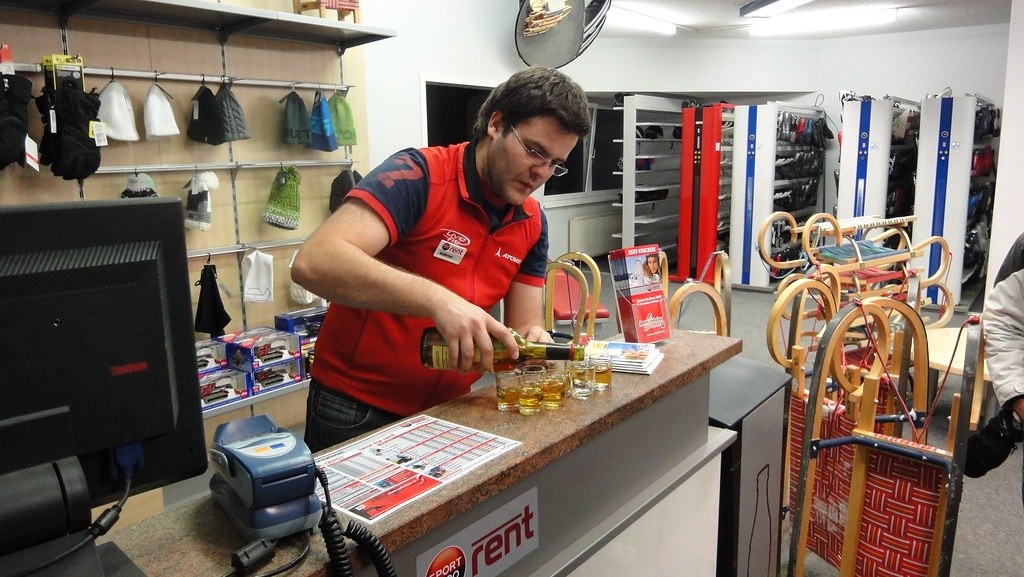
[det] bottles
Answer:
[420,327,584,372]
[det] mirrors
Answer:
[514,0,612,70]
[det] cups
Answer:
[496,354,612,416]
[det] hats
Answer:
[184,171,219,230]
[262,166,302,230]
[215,85,251,141]
[328,92,357,146]
[144,84,180,142]
[307,90,338,152]
[279,92,312,145]
[187,86,225,146]
[96,82,140,142]
[193,266,232,333]
[329,170,363,213]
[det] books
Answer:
[583,241,674,376]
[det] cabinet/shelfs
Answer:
[908,86,1001,313]
[611,94,693,264]
[668,103,735,287]
[728,100,826,293]
[834,89,921,291]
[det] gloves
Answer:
[0,74,100,180]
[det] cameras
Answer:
[960,406,1024,478]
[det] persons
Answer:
[962,228,1024,479]
[642,253,659,285]
[288,66,593,455]
[980,263,1024,504]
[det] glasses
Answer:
[510,124,568,177]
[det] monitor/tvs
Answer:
[0,197,209,577]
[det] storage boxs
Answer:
[226,357,304,397]
[274,307,329,345]
[197,368,252,411]
[211,326,301,372]
[195,339,229,376]
[300,342,318,380]
